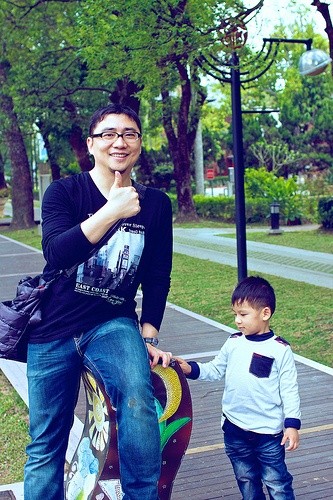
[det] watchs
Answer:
[142,336,159,347]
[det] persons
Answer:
[24,104,172,500]
[172,275,301,500]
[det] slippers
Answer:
[90,131,141,141]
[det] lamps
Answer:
[270,199,281,230]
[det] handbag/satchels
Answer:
[1,272,50,364]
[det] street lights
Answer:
[190,1,332,283]
